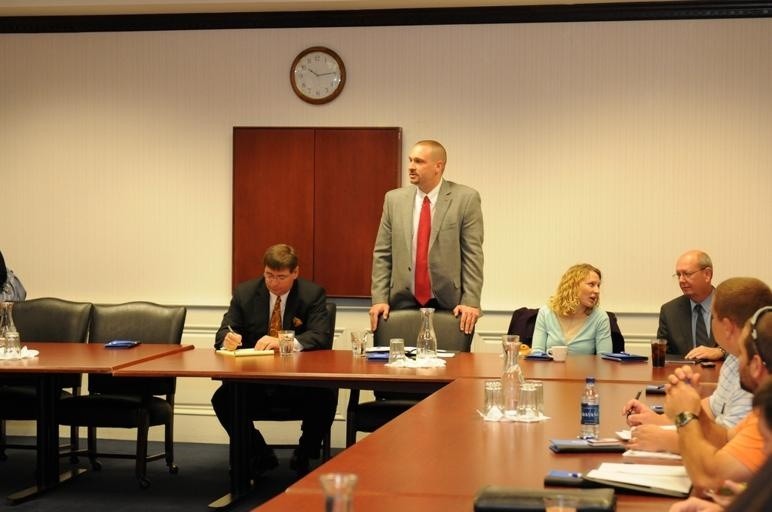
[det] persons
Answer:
[620,277,772,451]
[208,242,340,487]
[531,263,613,357]
[661,307,772,499]
[666,379,772,511]
[366,140,484,336]
[657,250,730,359]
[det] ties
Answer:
[415,196,432,306]
[695,304,709,347]
[269,296,282,352]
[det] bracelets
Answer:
[718,343,727,358]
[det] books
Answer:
[474,486,619,512]
[523,351,553,361]
[664,354,704,365]
[215,346,275,359]
[543,469,626,493]
[645,384,666,394]
[105,339,142,349]
[589,459,695,502]
[547,436,625,453]
[365,343,458,362]
[602,349,649,363]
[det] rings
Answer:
[631,436,637,443]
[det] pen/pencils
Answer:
[228,325,243,347]
[627,390,641,415]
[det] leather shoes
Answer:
[289,456,324,474]
[228,448,279,477]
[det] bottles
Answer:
[579,374,601,441]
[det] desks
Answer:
[251,375,719,512]
[1,338,194,507]
[114,345,726,509]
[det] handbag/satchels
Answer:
[0,270,26,302]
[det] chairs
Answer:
[509,309,625,352]
[228,304,338,485]
[344,306,477,469]
[66,295,187,481]
[0,295,95,480]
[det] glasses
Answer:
[672,267,705,279]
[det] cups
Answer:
[546,345,570,363]
[515,380,547,418]
[0,300,16,359]
[500,335,527,419]
[3,332,21,359]
[388,338,405,367]
[650,338,667,369]
[320,475,358,512]
[415,307,440,364]
[276,328,295,357]
[349,330,369,357]
[480,379,506,419]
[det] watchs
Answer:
[674,411,698,430]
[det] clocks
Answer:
[289,46,347,106]
